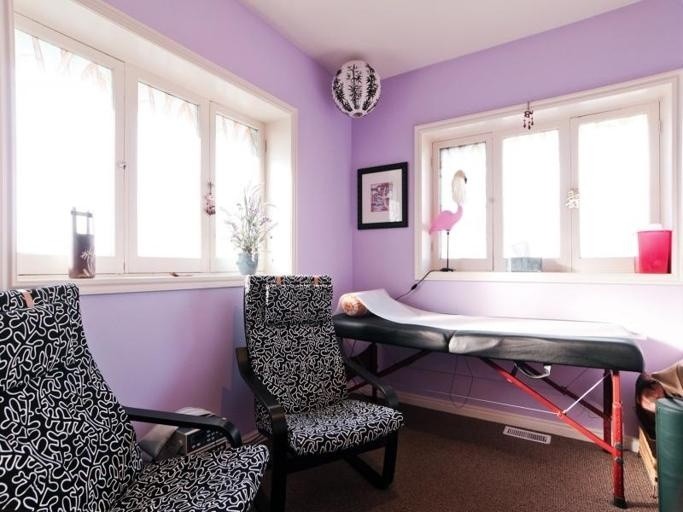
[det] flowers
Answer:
[224,184,276,262]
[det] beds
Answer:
[332,313,643,508]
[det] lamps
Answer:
[332,59,381,119]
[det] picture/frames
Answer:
[358,162,408,230]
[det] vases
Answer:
[238,254,257,274]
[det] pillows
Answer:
[0,302,75,393]
[341,295,371,317]
[265,285,333,325]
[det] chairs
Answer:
[236,274,404,512]
[0,283,270,512]
